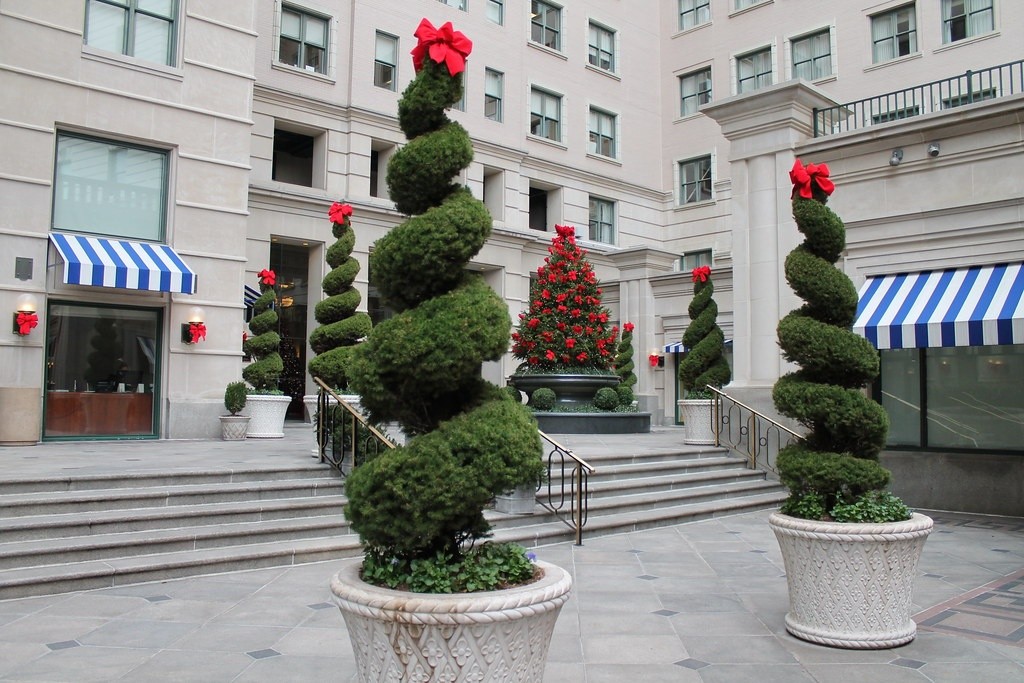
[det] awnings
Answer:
[45,234,198,296]
[662,339,735,353]
[844,259,1024,350]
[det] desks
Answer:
[43,392,152,436]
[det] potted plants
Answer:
[217,381,251,441]
[84,302,125,392]
[302,201,372,457]
[327,16,572,682]
[766,156,934,650]
[676,264,730,445]
[240,268,292,437]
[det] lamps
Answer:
[12,293,37,335]
[181,306,206,344]
[650,348,664,368]
